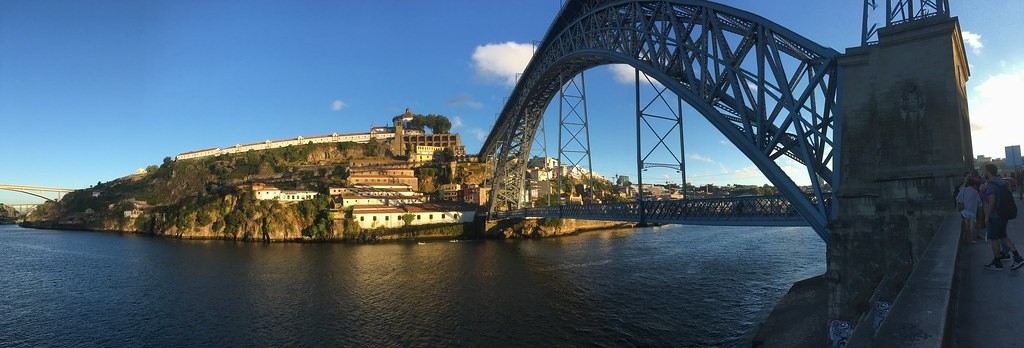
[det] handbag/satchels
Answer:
[957,202,964,211]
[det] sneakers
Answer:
[984,262,1003,271]
[1010,256,1024,270]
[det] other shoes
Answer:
[967,239,978,244]
[1000,251,1010,261]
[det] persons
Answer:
[953,169,1024,261]
[813,195,832,206]
[984,164,1024,271]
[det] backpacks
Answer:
[986,180,1017,219]
